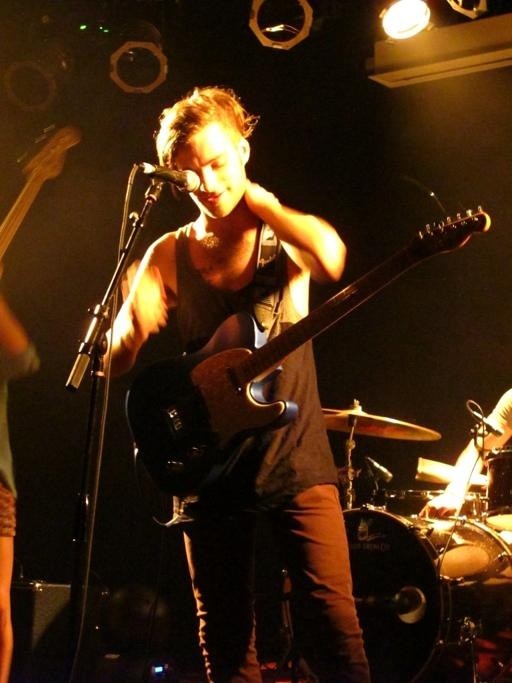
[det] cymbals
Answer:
[321,405,442,441]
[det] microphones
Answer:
[474,411,504,439]
[137,161,199,193]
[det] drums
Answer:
[282,503,512,683]
[484,446,512,532]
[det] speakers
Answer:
[11,580,74,683]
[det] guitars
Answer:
[124,206,492,495]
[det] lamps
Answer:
[0,19,169,115]
[247,1,313,53]
[366,0,433,40]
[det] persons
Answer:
[418,388,512,521]
[98,80,379,683]
[0,262,47,682]
